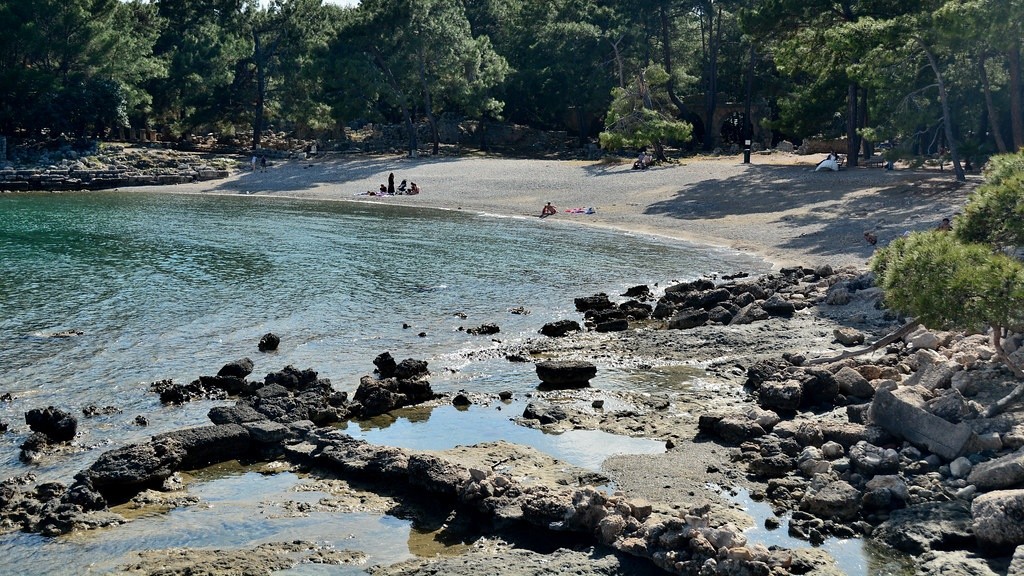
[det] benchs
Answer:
[834,158,844,171]
[865,155,884,168]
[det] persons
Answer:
[252,156,257,175]
[631,152,653,170]
[815,150,840,172]
[260,155,268,173]
[397,179,408,195]
[863,231,877,245]
[938,218,953,231]
[543,202,559,214]
[407,182,420,195]
[380,184,387,193]
[388,173,395,195]
